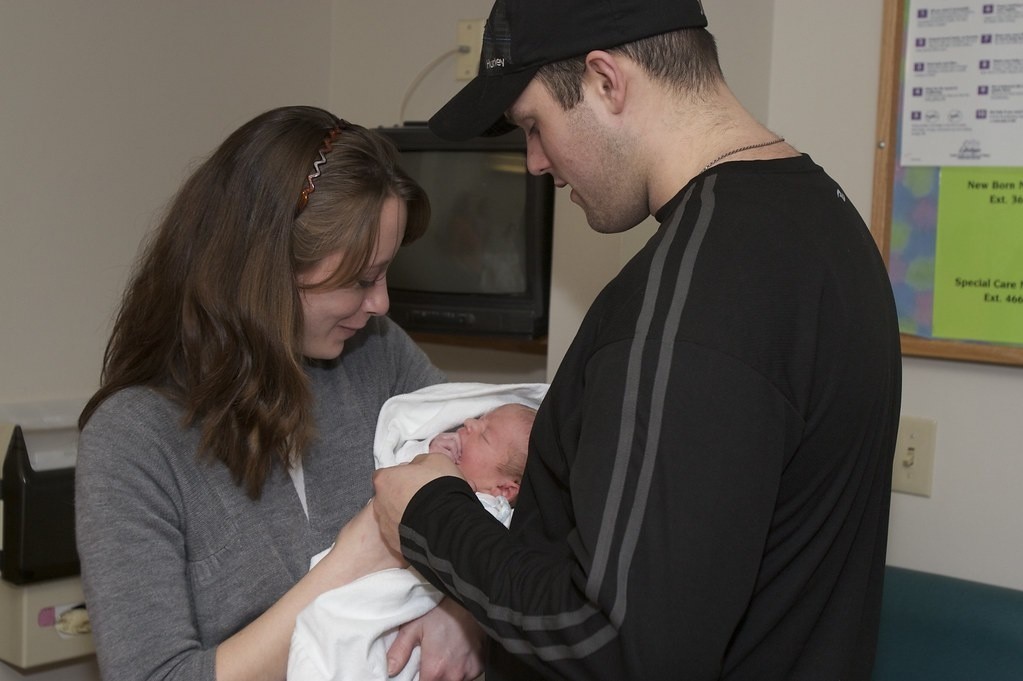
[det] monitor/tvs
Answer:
[367,120,555,340]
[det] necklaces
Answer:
[699,138,785,174]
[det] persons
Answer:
[287,402,538,681]
[371,0,901,681]
[73,105,491,680]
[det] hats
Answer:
[428,0,707,142]
